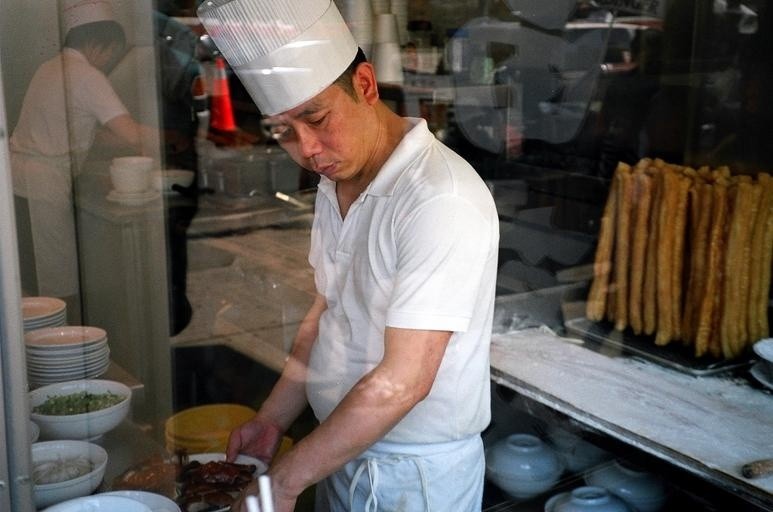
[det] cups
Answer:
[339,0,405,86]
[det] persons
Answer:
[152,11,208,337]
[225,49,499,512]
[9,20,158,322]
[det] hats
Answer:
[63,0,116,41]
[196,1,359,119]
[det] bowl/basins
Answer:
[106,155,193,206]
[29,439,107,506]
[43,490,178,512]
[483,391,689,512]
[28,380,130,441]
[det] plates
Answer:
[747,339,772,389]
[113,452,266,510]
[20,295,112,387]
[166,402,293,473]
